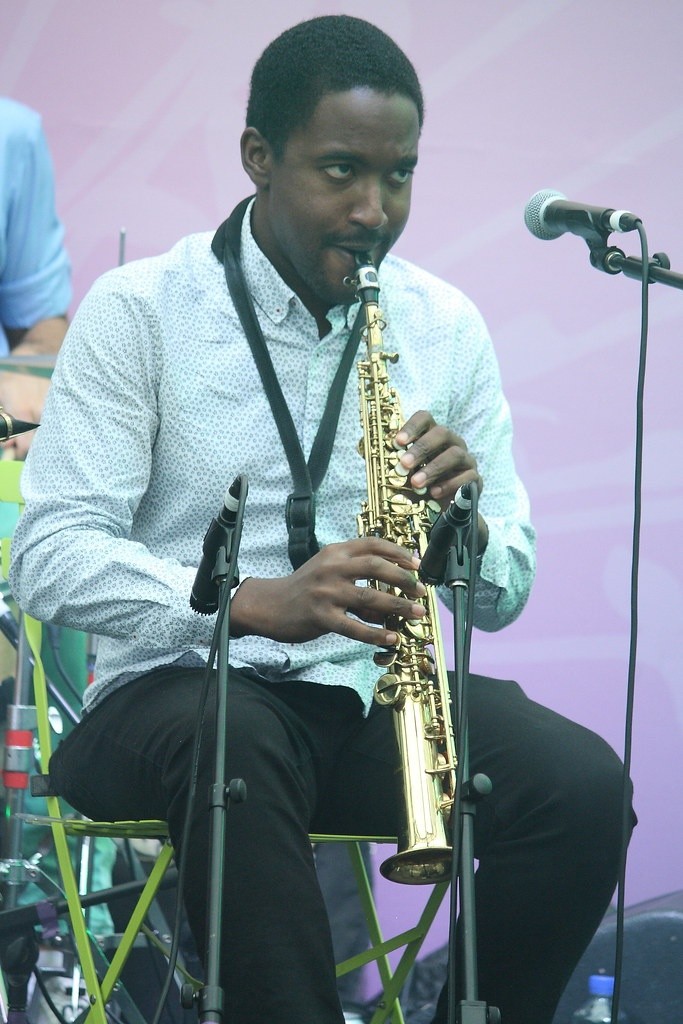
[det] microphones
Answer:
[522,188,641,240]
[189,474,248,615]
[417,480,474,586]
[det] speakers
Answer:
[550,889,682,1024]
[102,837,375,1023]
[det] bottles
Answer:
[571,974,628,1024]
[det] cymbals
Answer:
[0,355,58,379]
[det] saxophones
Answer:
[352,255,467,886]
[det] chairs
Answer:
[0,455,448,1024]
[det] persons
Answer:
[0,93,73,460]
[10,17,639,1023]
